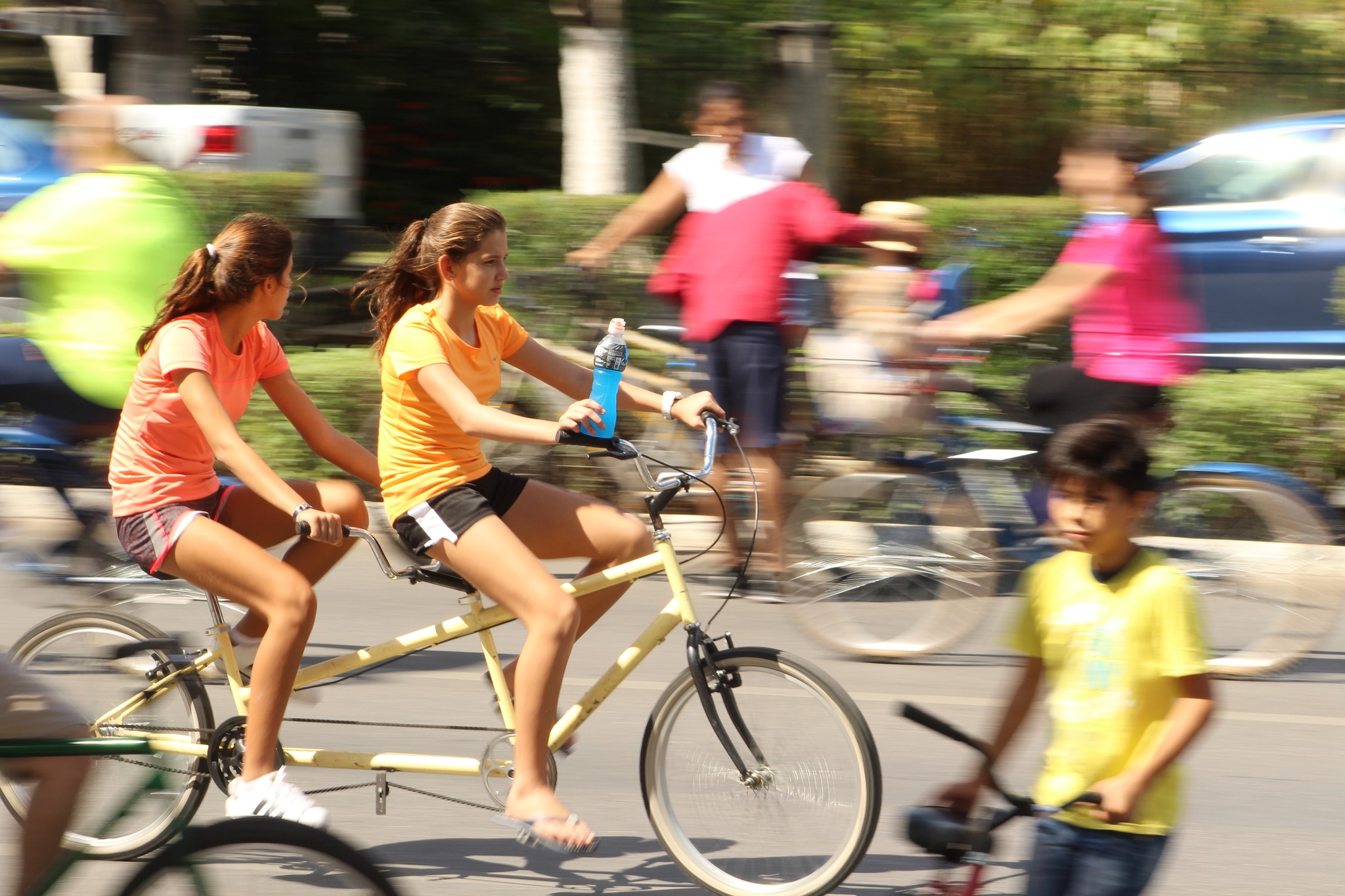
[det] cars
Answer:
[1127,108,1345,374]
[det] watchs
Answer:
[662,391,683,420]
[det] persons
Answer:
[930,423,1216,896]
[0,95,209,447]
[106,212,382,830]
[877,121,1201,536]
[560,85,949,586]
[351,202,726,855]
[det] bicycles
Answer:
[0,385,885,895]
[892,701,1108,896]
[532,252,1345,683]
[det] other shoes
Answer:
[721,558,774,591]
[777,564,859,594]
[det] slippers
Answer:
[486,672,576,758]
[491,811,598,852]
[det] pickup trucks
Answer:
[3,73,363,289]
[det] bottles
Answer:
[579,317,628,438]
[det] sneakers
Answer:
[225,766,327,828]
[193,637,261,681]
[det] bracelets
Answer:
[292,503,313,523]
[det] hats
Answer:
[858,199,931,253]
